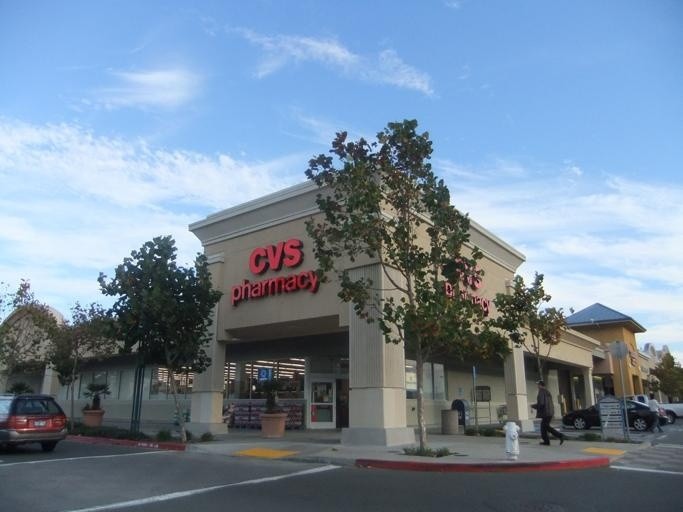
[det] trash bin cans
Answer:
[441,409,458,434]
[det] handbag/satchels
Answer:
[659,408,666,416]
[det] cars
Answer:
[561,397,668,432]
[0,393,68,452]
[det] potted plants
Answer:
[256,381,288,437]
[81,384,110,428]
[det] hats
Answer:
[536,380,544,384]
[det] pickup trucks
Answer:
[624,395,683,425]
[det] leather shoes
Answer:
[560,435,565,445]
[540,442,550,445]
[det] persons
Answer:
[649,393,663,433]
[531,380,566,445]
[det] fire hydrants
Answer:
[503,421,521,461]
